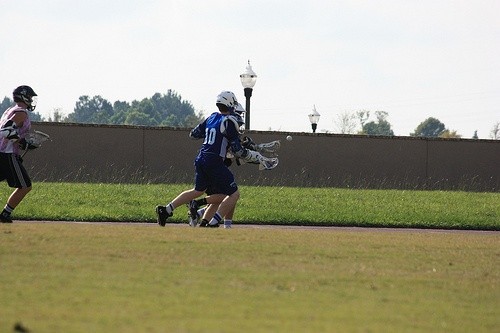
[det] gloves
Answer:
[242,135,265,156]
[245,149,262,163]
[18,138,41,150]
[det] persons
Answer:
[156,89,280,228]
[1,84,37,223]
[187,102,252,227]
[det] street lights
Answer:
[308,105,321,133]
[240,60,258,130]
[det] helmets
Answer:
[235,103,247,112]
[13,85,38,102]
[216,90,238,110]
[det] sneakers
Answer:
[186,199,220,227]
[0,213,13,223]
[156,205,169,226]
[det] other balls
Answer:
[286,136,292,142]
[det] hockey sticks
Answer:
[257,155,279,171]
[257,141,281,153]
